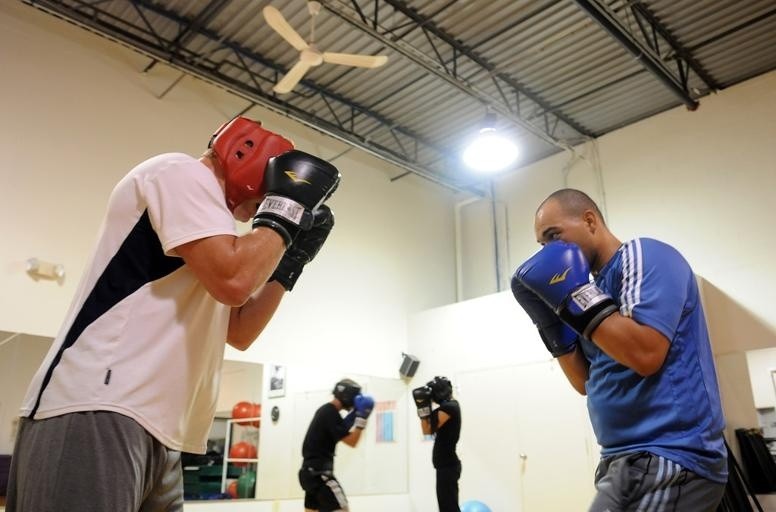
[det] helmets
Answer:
[332,379,361,411]
[426,376,452,405]
[208,114,295,215]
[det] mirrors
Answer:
[0,331,408,507]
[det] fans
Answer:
[262,0,389,94]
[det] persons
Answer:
[5,113,344,511]
[299,378,374,510]
[508,187,730,511]
[411,375,463,510]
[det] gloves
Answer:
[267,204,333,293]
[514,239,619,342]
[352,394,374,430]
[413,385,433,419]
[510,273,579,358]
[252,149,341,251]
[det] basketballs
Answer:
[233,402,254,425]
[231,443,256,466]
[229,482,238,498]
[249,405,261,428]
